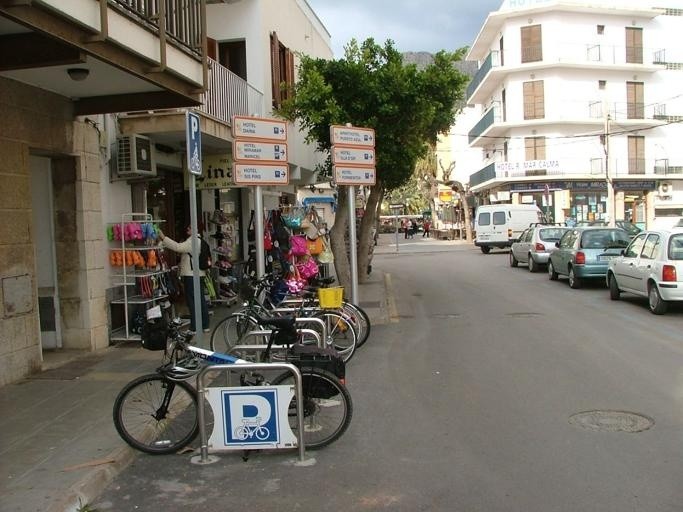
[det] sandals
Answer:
[139,274,168,298]
[108,250,158,267]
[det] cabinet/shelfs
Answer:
[205,211,239,308]
[107,213,192,342]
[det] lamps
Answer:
[69,68,90,82]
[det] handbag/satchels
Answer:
[300,353,345,400]
[243,206,334,293]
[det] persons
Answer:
[159,221,210,334]
[396,216,431,239]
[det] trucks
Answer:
[473,202,549,254]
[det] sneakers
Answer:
[184,328,210,335]
[210,209,238,299]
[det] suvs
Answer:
[507,221,572,270]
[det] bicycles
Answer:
[110,307,353,452]
[211,274,370,368]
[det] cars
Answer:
[546,224,631,290]
[602,229,682,314]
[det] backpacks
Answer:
[187,236,212,270]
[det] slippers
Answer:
[107,222,161,242]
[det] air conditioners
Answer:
[114,133,157,178]
[658,181,673,197]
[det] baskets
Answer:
[318,286,344,309]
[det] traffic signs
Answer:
[231,159,290,187]
[230,138,289,163]
[387,203,404,209]
[330,144,376,167]
[331,164,376,186]
[229,114,287,142]
[329,124,377,148]
